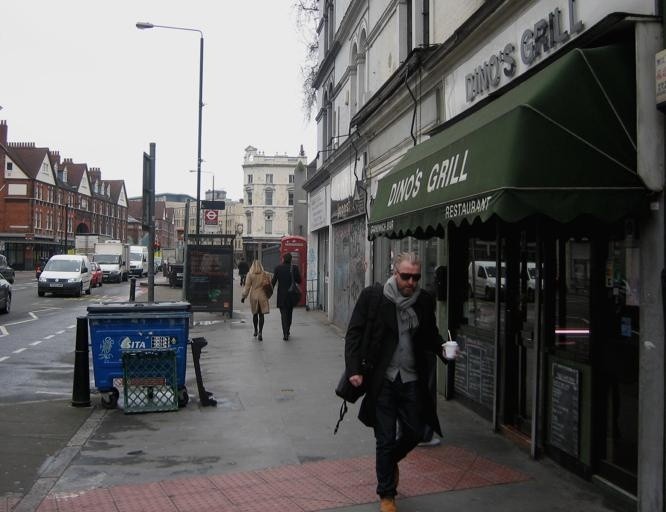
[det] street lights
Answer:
[189,168,214,200]
[137,22,204,244]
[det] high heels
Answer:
[254,329,258,338]
[258,333,262,341]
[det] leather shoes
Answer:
[287,332,290,335]
[283,337,288,340]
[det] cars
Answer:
[87,260,101,287]
[153,259,168,274]
[0,277,10,313]
[0,253,14,284]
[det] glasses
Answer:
[395,267,421,281]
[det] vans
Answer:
[129,245,147,278]
[36,254,91,297]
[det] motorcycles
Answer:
[34,265,41,279]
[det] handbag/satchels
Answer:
[289,277,302,302]
[263,283,273,299]
[333,359,375,402]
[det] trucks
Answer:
[467,260,506,299]
[94,241,128,283]
[519,262,543,301]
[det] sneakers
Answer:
[418,438,439,447]
[380,499,397,512]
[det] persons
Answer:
[271,252,302,342]
[344,252,460,512]
[240,259,274,341]
[238,257,250,286]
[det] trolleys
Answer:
[86,302,191,409]
